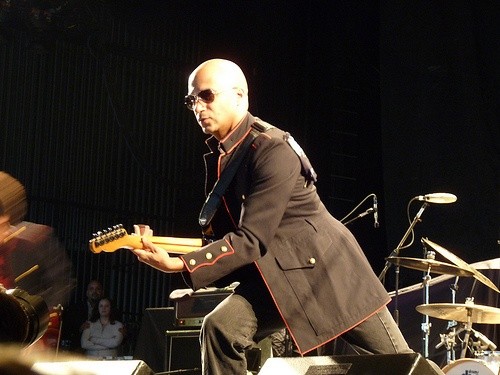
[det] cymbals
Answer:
[386,256,476,275]
[422,237,500,294]
[469,258,500,270]
[415,304,500,324]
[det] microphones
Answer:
[373,195,379,229]
[415,192,457,204]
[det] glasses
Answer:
[184,87,245,110]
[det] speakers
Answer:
[257,351,439,375]
[0,343,157,374]
[163,330,201,375]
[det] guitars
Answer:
[89,222,242,288]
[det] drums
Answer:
[442,352,500,375]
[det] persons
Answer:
[133,59,444,375]
[61,279,108,349]
[81,296,124,360]
